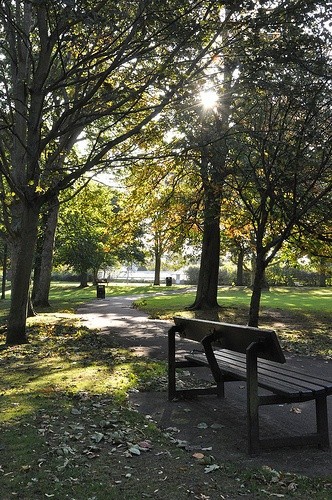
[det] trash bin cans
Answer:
[166,276,173,287]
[97,285,106,300]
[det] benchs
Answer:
[166,316,332,458]
